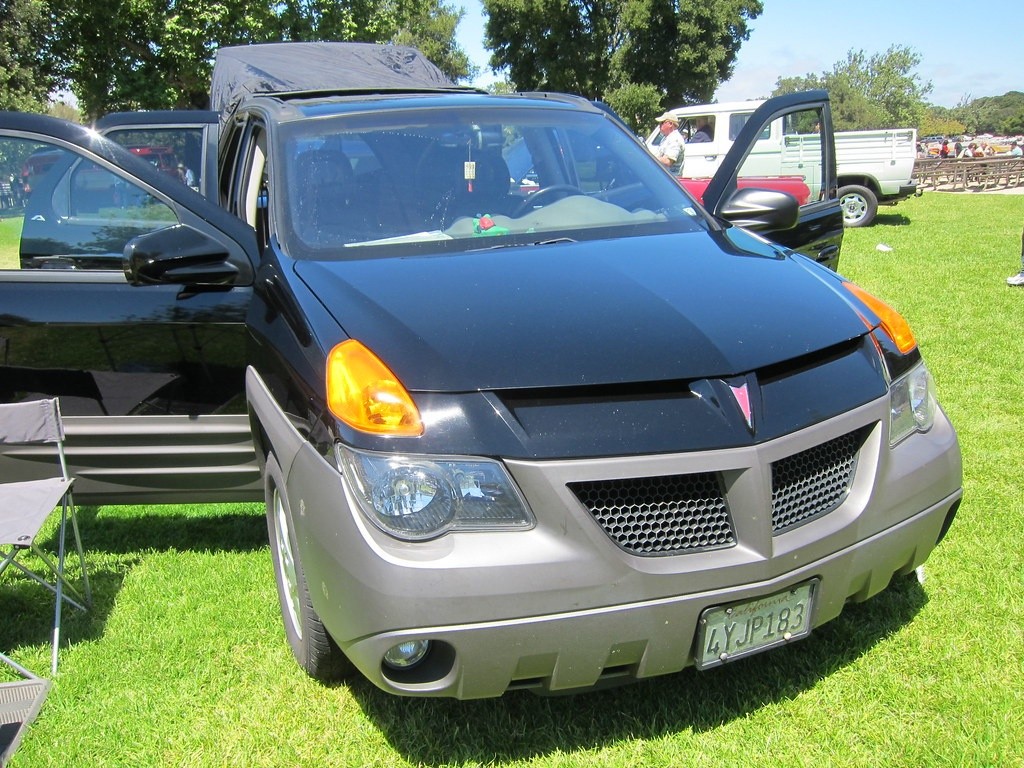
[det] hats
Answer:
[655,112,678,122]
[1010,141,1017,145]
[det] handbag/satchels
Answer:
[940,150,947,155]
[962,149,970,162]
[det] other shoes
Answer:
[1007,273,1024,285]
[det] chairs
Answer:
[0,396,92,682]
[439,149,533,226]
[295,150,383,247]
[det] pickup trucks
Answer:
[465,126,810,215]
[642,98,926,231]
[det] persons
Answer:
[916,139,995,180]
[689,116,714,143]
[1007,226,1024,285]
[1006,137,1024,165]
[0,164,32,208]
[812,123,820,134]
[655,112,686,177]
[177,163,195,187]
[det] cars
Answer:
[27,144,181,203]
[0,40,966,702]
